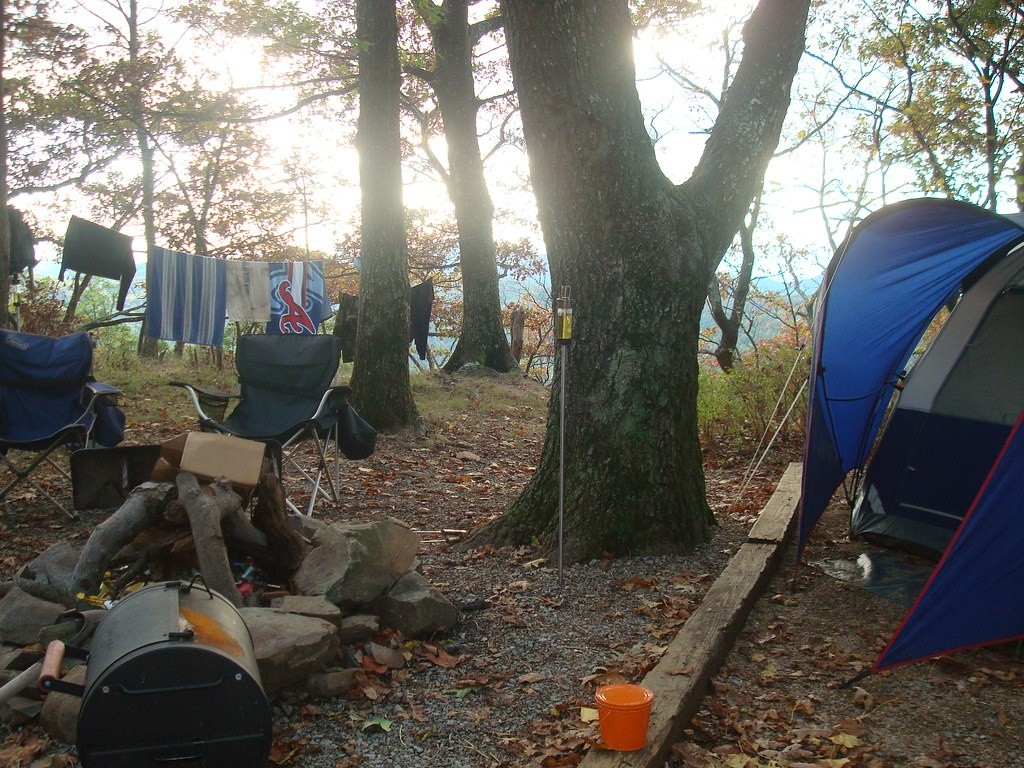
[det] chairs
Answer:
[0,328,126,522]
[167,334,378,518]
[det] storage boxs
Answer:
[151,430,265,514]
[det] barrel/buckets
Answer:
[595,684,655,751]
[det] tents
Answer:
[792,197,1024,673]
